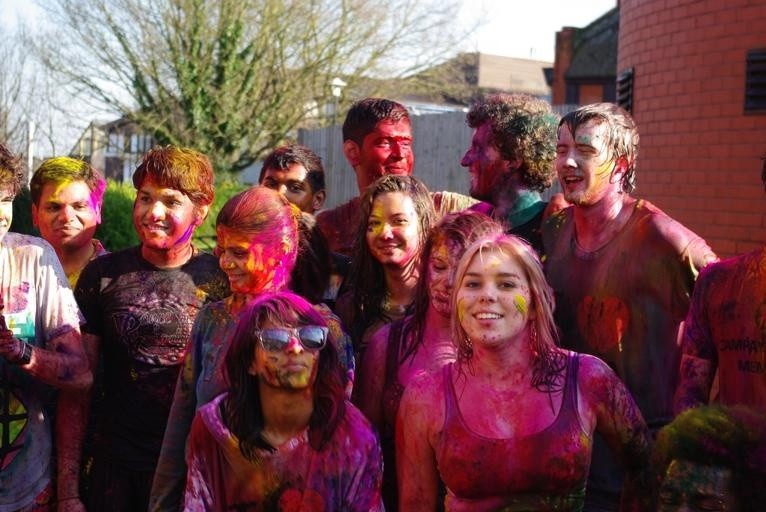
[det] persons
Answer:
[536,97,720,511]
[456,90,561,260]
[182,293,389,512]
[672,159,765,472]
[642,402,765,512]
[287,95,485,311]
[258,145,328,219]
[50,142,227,511]
[0,142,94,512]
[334,174,439,348]
[394,231,665,511]
[30,155,108,295]
[350,207,500,511]
[145,185,357,512]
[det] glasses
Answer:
[252,325,330,353]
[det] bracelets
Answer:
[11,338,33,366]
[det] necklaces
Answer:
[190,242,195,258]
